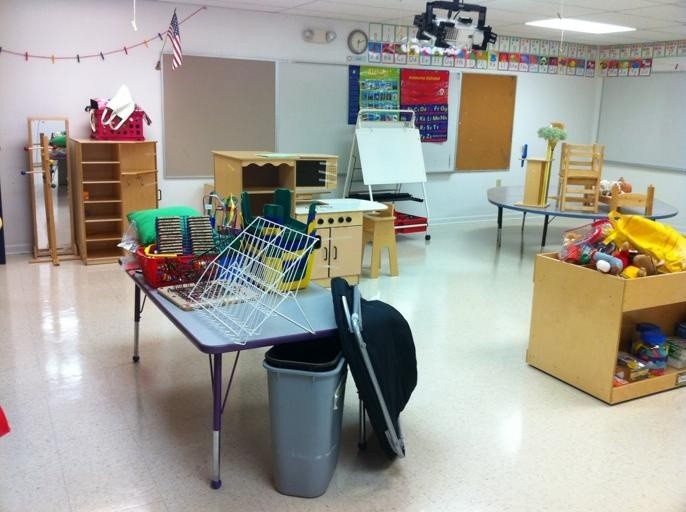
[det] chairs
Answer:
[361,202,399,283]
[556,146,606,213]
[558,139,605,216]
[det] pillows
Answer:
[127,203,207,245]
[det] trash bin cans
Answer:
[262,336,349,498]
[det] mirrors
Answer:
[25,114,77,265]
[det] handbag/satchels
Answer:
[608,208,686,271]
[100,84,137,131]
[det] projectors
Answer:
[423,17,485,47]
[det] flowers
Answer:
[536,123,569,146]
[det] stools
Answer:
[237,186,322,293]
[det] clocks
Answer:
[346,28,369,55]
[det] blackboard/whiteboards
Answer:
[596,70,686,172]
[354,128,427,185]
[274,59,462,176]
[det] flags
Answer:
[168,14,183,72]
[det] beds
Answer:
[598,182,656,219]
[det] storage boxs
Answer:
[662,334,686,369]
[133,244,221,288]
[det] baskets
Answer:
[93,108,145,141]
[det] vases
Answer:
[543,146,556,208]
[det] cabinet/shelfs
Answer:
[209,148,297,228]
[524,245,686,406]
[70,138,162,266]
[290,197,389,289]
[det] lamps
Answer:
[302,28,338,45]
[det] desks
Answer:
[514,158,556,208]
[487,181,681,256]
[118,251,370,492]
